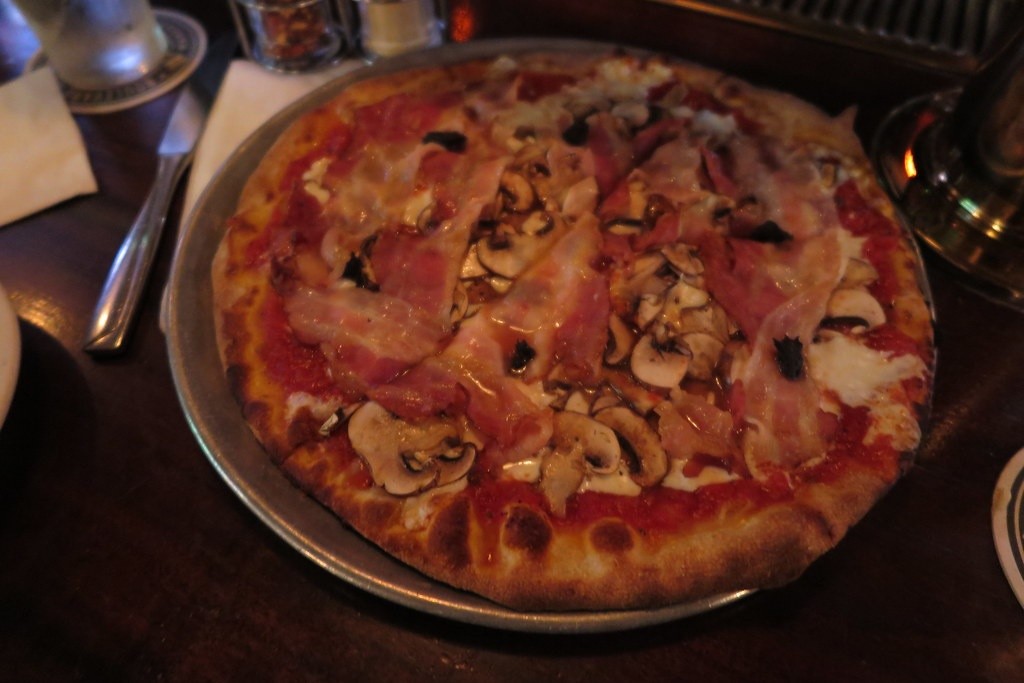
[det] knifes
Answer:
[82,83,207,354]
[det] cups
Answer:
[4,0,166,92]
[903,24,1024,245]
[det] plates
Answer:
[876,92,1024,312]
[22,11,209,114]
[164,37,937,632]
[991,447,1024,610]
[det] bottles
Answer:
[338,0,451,67]
[228,0,345,74]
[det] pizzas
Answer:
[208,53,934,615]
